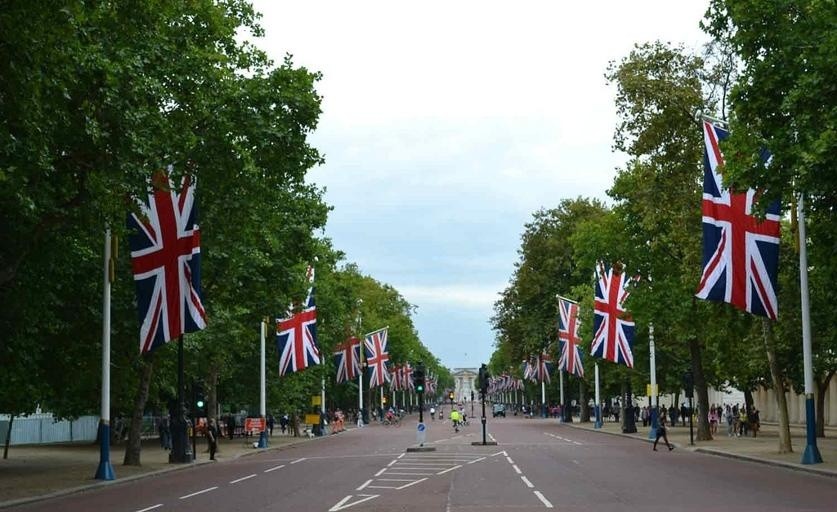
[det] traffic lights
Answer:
[471,392,474,400]
[684,371,694,398]
[484,368,489,392]
[414,366,424,393]
[448,390,454,401]
[196,381,206,414]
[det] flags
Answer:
[592,260,645,369]
[559,299,591,381]
[125,154,214,340]
[696,123,781,324]
[276,257,320,376]
[336,318,553,389]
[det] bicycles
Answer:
[429,414,435,422]
[454,416,470,432]
[382,416,402,428]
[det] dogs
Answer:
[306,429,315,439]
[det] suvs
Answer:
[492,405,506,418]
